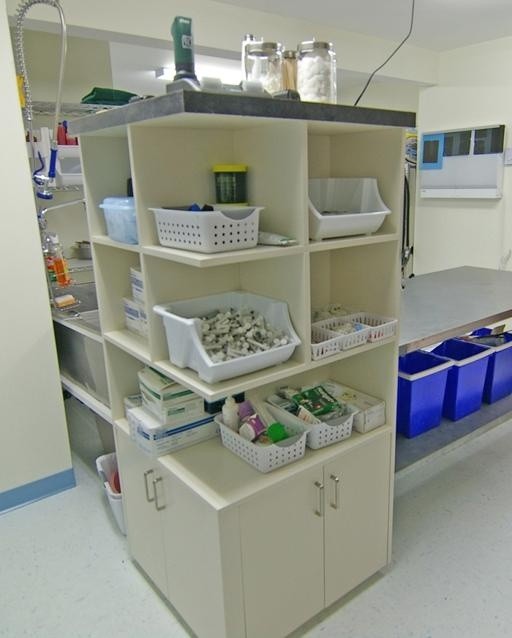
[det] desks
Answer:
[395,265,512,498]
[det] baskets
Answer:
[148,205,266,253]
[214,385,360,473]
[311,313,398,361]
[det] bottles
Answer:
[241,34,338,104]
[224,396,239,432]
[212,164,248,203]
[57,123,65,145]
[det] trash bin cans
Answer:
[399,326,511,444]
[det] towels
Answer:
[81,87,138,105]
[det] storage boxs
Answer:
[96,452,127,536]
[397,349,455,440]
[127,366,222,457]
[421,337,495,422]
[98,196,139,244]
[455,327,512,404]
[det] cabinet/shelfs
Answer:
[67,90,416,638]
